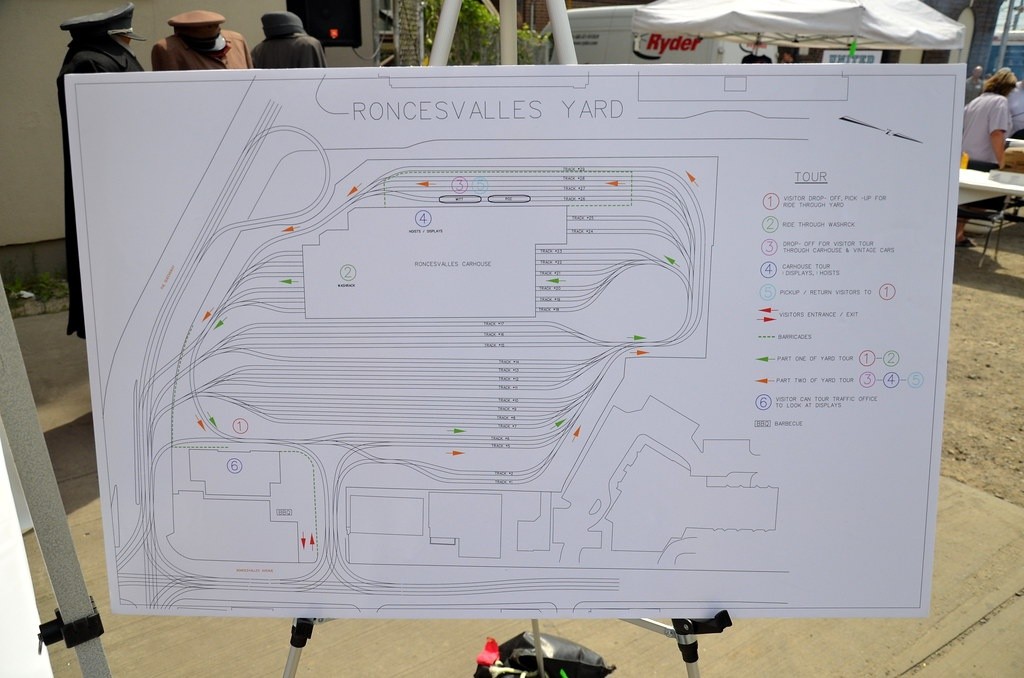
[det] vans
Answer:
[534,4,780,65]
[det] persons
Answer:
[1005,74,1024,142]
[965,64,987,105]
[954,66,1017,246]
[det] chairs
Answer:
[958,159,1010,269]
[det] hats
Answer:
[169,10,226,51]
[59,1,148,43]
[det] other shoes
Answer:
[956,235,975,246]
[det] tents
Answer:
[559,0,963,70]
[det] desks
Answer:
[957,169,1024,206]
